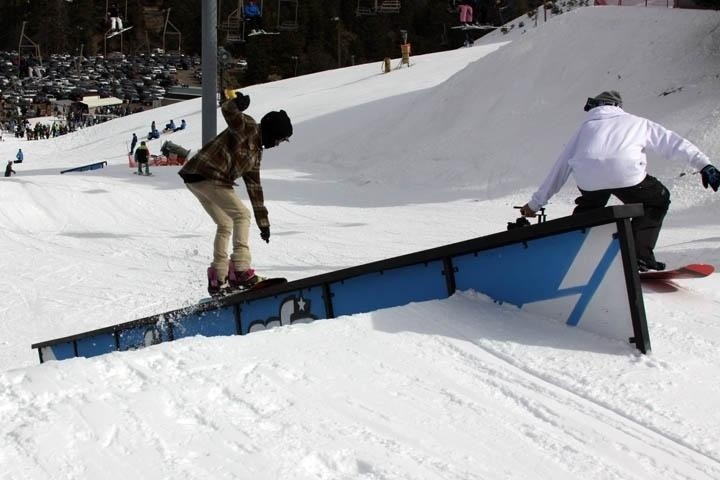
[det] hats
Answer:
[260,108,293,149]
[594,91,623,107]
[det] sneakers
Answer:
[638,258,666,272]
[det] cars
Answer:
[0,46,248,123]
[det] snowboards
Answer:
[133,171,155,177]
[640,264,714,278]
[200,278,287,302]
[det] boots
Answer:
[209,267,246,298]
[228,260,264,287]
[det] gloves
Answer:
[699,164,720,192]
[234,89,251,111]
[259,225,271,243]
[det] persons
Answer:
[4,103,135,140]
[5,160,16,178]
[130,132,139,154]
[14,148,24,164]
[242,0,266,34]
[522,89,719,273]
[106,2,126,33]
[134,141,153,175]
[179,91,292,296]
[451,3,475,25]
[148,119,186,140]
